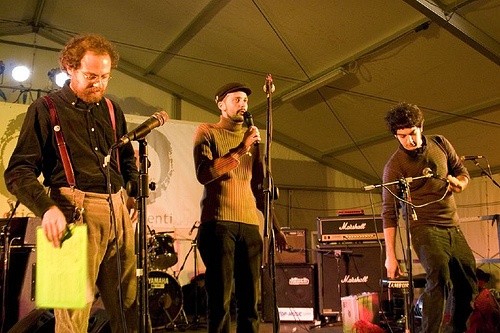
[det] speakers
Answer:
[317,244,390,315]
[0,216,113,333]
[262,228,308,265]
[259,265,318,323]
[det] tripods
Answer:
[176,236,206,331]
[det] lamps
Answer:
[47,67,71,88]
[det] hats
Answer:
[215,82,252,104]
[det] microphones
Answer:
[460,156,484,161]
[243,111,260,147]
[423,167,449,183]
[112,110,169,149]
[7,199,14,210]
[188,221,197,236]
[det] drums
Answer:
[147,234,178,269]
[137,271,183,326]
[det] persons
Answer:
[381,100,477,333]
[3,33,140,333]
[194,83,289,333]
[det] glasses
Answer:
[74,71,111,81]
